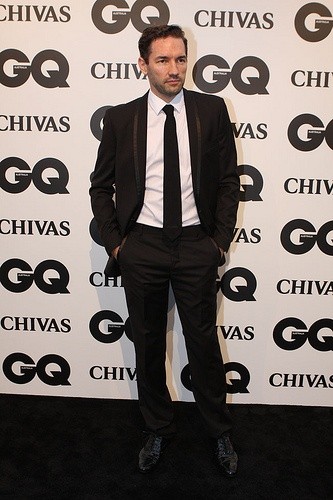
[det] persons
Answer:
[91,24,240,478]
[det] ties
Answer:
[161,105,182,240]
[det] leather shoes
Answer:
[138,434,163,474]
[212,429,238,478]
